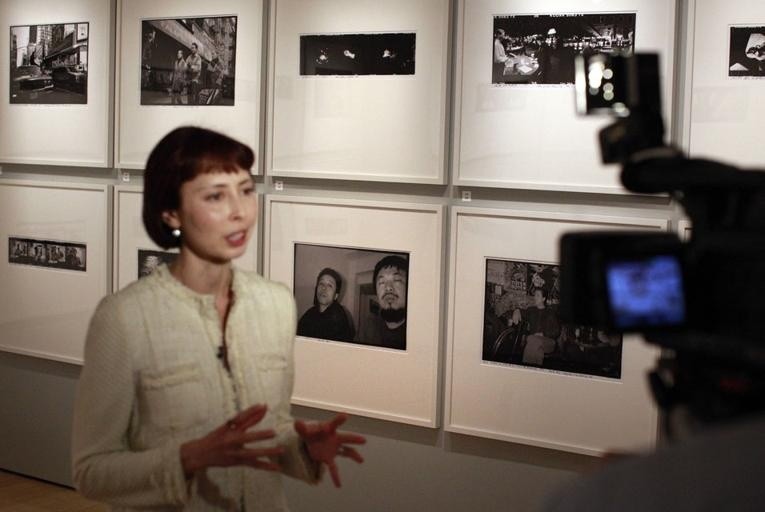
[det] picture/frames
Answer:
[112,187,258,293]
[444,204,668,456]
[0,0,114,170]
[114,2,262,176]
[263,1,451,188]
[0,178,112,368]
[258,195,440,430]
[677,220,695,240]
[452,2,676,196]
[678,0,765,169]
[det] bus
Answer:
[40,44,87,91]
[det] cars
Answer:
[11,65,55,101]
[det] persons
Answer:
[186,43,202,105]
[346,256,408,350]
[70,125,366,504]
[493,24,633,75]
[169,49,188,104]
[296,267,354,342]
[512,288,561,367]
[30,52,39,66]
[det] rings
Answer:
[228,417,238,430]
